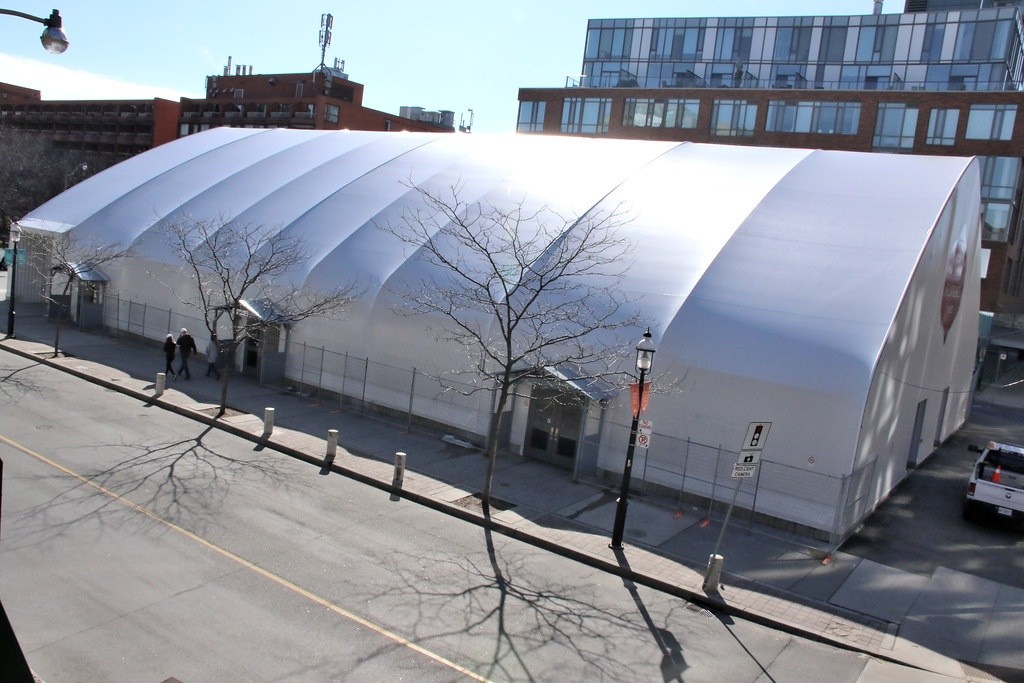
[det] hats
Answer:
[167,334,172,337]
[180,328,187,333]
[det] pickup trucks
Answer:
[963,441,1024,525]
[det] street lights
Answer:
[5,222,23,340]
[606,327,661,548]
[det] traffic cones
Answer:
[991,466,1002,484]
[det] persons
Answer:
[176,327,197,379]
[206,334,221,380]
[164,334,177,381]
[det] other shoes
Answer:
[172,375,177,381]
[205,373,210,376]
[177,370,181,375]
[184,377,191,380]
[217,375,221,379]
